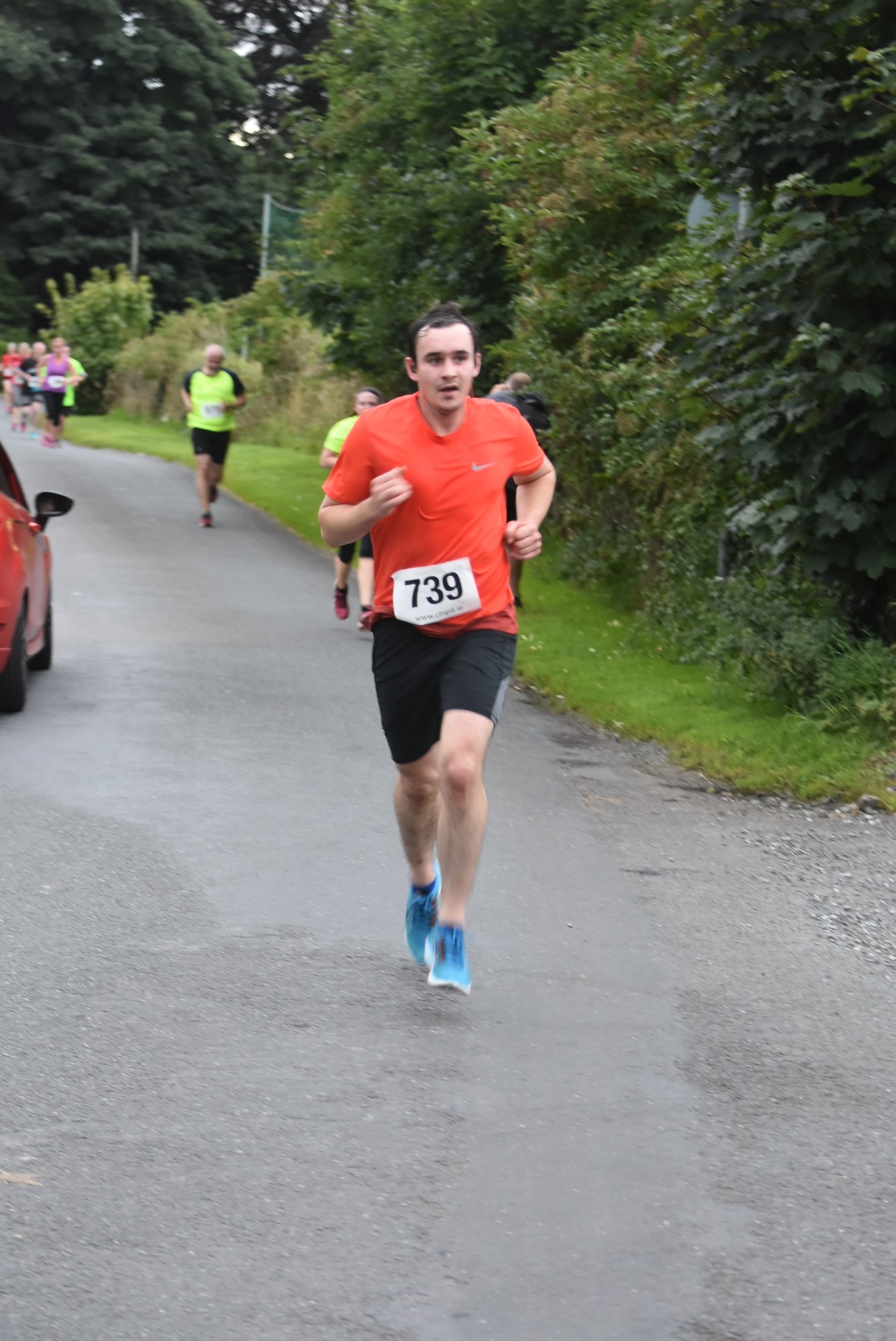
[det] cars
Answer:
[0,444,76,719]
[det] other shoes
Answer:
[201,513,211,527]
[210,486,217,504]
[11,422,18,431]
[20,423,26,431]
[5,405,11,412]
[42,432,63,448]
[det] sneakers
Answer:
[423,921,472,997]
[357,608,374,633]
[405,859,443,965]
[333,580,350,620]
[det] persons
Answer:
[181,344,246,528]
[482,371,554,607]
[0,337,89,447]
[320,307,556,996]
[317,386,383,629]
